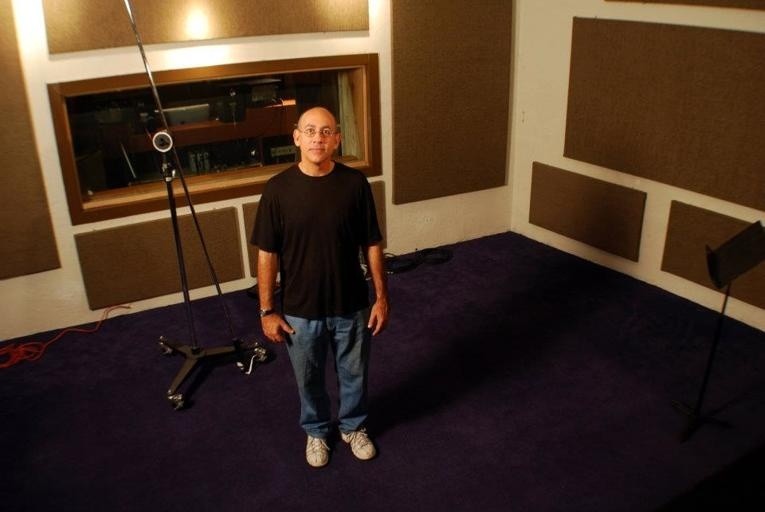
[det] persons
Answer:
[250,106,388,468]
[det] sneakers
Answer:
[306,435,331,467]
[341,427,376,460]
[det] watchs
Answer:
[259,308,274,318]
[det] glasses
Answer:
[297,128,338,137]
